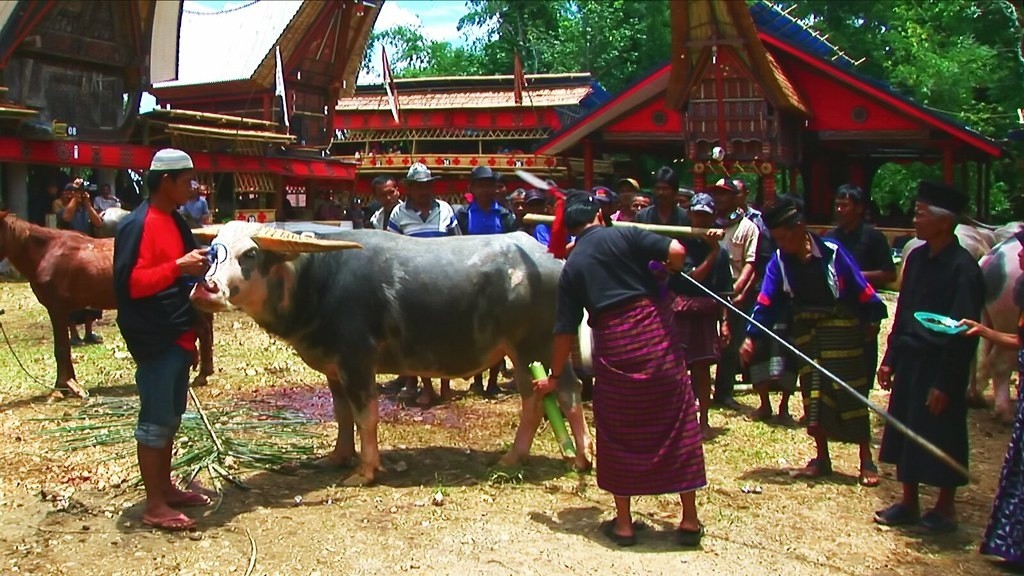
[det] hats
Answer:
[690,193,714,214]
[525,189,544,206]
[618,178,639,192]
[708,177,740,194]
[916,181,969,216]
[761,196,798,230]
[66,184,82,191]
[191,180,199,189]
[149,148,193,170]
[592,187,616,202]
[564,190,612,227]
[470,164,494,182]
[401,162,442,184]
[733,179,748,191]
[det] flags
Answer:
[272,45,289,128]
[378,44,401,123]
[513,48,528,107]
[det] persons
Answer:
[818,183,897,295]
[531,188,708,547]
[873,182,986,532]
[738,197,888,487]
[28,166,794,430]
[114,148,212,532]
[955,227,1024,562]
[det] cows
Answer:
[899,216,1024,429]
[0,209,216,403]
[189,217,595,486]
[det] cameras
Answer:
[80,181,97,191]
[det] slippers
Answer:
[802,457,832,478]
[168,491,211,506]
[70,336,86,346]
[84,334,103,343]
[602,517,636,545]
[873,503,917,525]
[680,518,704,546]
[143,512,196,531]
[911,512,957,534]
[860,461,878,486]
